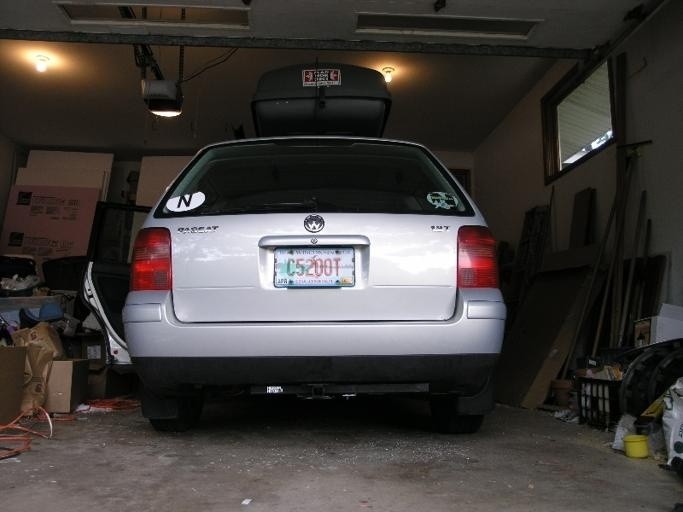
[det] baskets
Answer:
[570,375,621,427]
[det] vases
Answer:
[550,379,574,406]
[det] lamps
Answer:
[382,66,396,83]
[140,65,183,118]
[32,53,49,74]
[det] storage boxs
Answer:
[0,346,27,426]
[44,358,88,413]
[72,337,106,371]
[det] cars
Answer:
[79,60,507,431]
